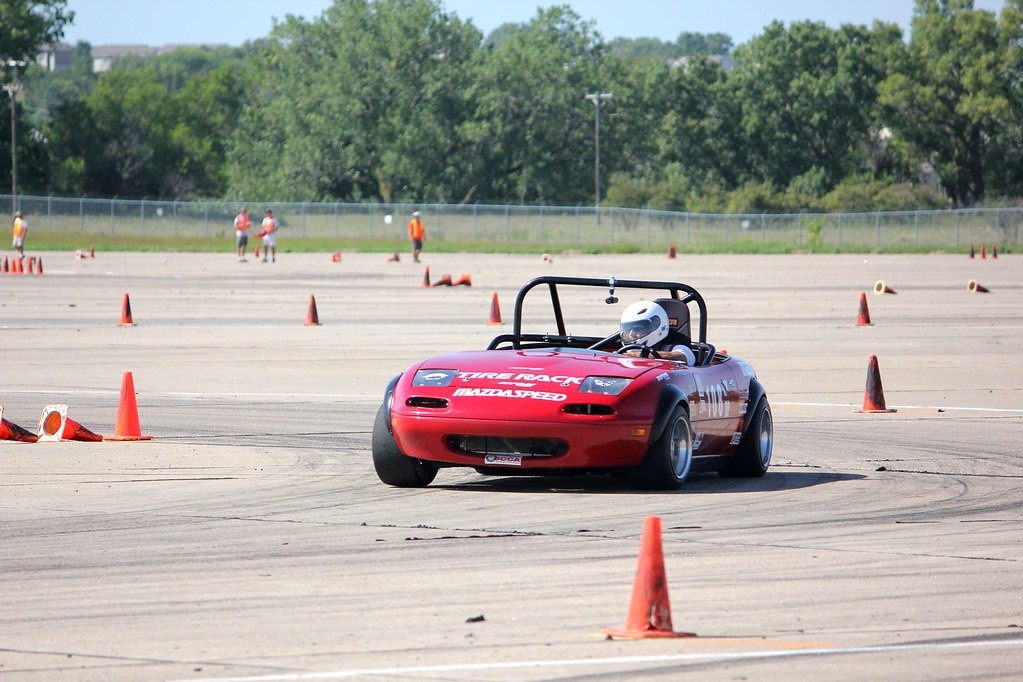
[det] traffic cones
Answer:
[873,280,898,296]
[88,245,95,259]
[854,291,875,326]
[1,255,9,272]
[114,292,137,327]
[76,250,86,260]
[990,242,999,260]
[36,403,103,442]
[385,252,399,262]
[979,244,988,260]
[24,256,33,274]
[966,279,989,295]
[9,257,16,273]
[0,404,39,443]
[482,292,506,325]
[419,265,431,287]
[101,370,152,441]
[432,273,452,287]
[302,293,323,327]
[599,516,698,639]
[331,251,343,264]
[851,353,900,412]
[34,257,44,275]
[452,273,472,287]
[967,243,975,260]
[665,243,677,260]
[16,256,23,274]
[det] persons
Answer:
[262,207,280,262]
[612,300,696,365]
[408,210,426,263]
[12,210,28,259]
[233,207,250,261]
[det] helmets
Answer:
[619,301,670,350]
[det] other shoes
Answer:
[271,256,275,262]
[239,256,248,262]
[261,256,267,262]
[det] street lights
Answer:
[2,83,24,217]
[583,90,615,227]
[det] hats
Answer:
[413,211,420,216]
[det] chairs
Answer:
[655,298,693,350]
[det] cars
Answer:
[372,274,776,488]
